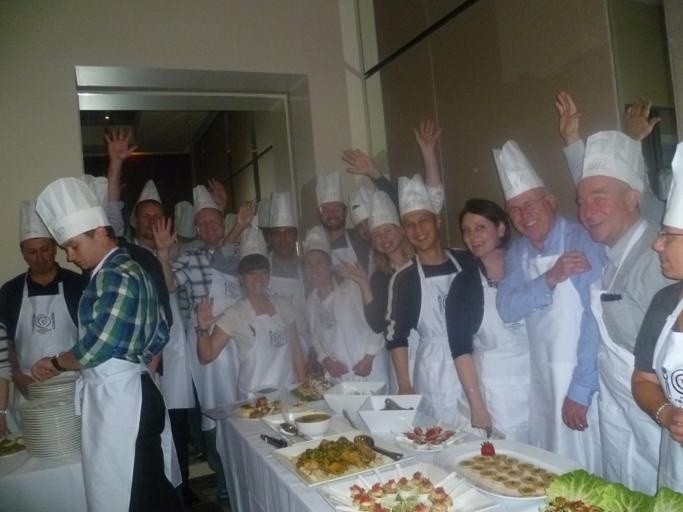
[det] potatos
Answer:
[480,441,494,457]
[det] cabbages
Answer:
[598,482,653,512]
[545,468,604,507]
[654,484,683,511]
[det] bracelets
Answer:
[656,402,671,424]
[465,388,482,394]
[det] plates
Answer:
[315,462,500,512]
[286,381,325,407]
[26,374,78,400]
[396,424,467,452]
[433,449,567,502]
[273,433,415,487]
[261,412,302,445]
[16,396,81,461]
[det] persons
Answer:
[194,165,242,244]
[618,94,664,227]
[313,149,399,278]
[292,222,392,393]
[211,190,319,382]
[0,196,89,399]
[553,89,675,494]
[493,137,604,471]
[444,197,534,445]
[170,198,200,245]
[331,118,444,273]
[630,139,683,497]
[0,317,15,441]
[150,184,245,500]
[195,246,308,400]
[384,171,473,395]
[33,176,182,512]
[330,191,416,335]
[100,125,202,506]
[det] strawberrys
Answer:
[480,440,495,457]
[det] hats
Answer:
[258,197,271,228]
[368,190,401,233]
[174,201,197,239]
[238,225,270,264]
[578,129,648,194]
[130,179,165,231]
[492,139,547,202]
[398,173,436,215]
[224,213,241,241]
[35,176,113,246]
[193,186,223,217]
[302,226,331,260]
[663,141,681,228]
[349,186,372,225]
[19,201,54,243]
[658,166,671,199]
[271,192,297,228]
[317,172,346,206]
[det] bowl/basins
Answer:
[324,380,386,413]
[357,393,426,441]
[254,386,281,403]
[295,411,331,437]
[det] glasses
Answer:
[652,231,679,241]
[507,190,553,216]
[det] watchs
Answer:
[196,327,211,336]
[49,352,66,373]
[0,407,10,415]
